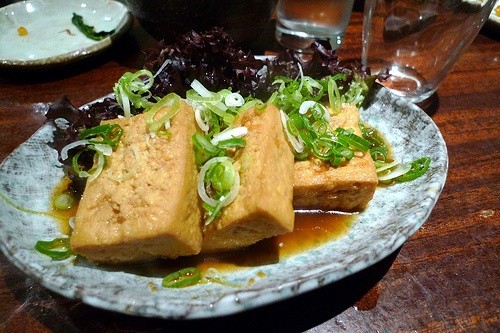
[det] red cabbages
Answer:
[45,27,392,196]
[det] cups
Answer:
[274,0,355,56]
[358,0,497,104]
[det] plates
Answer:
[0,0,136,67]
[1,52,448,321]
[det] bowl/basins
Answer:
[123,0,279,56]
